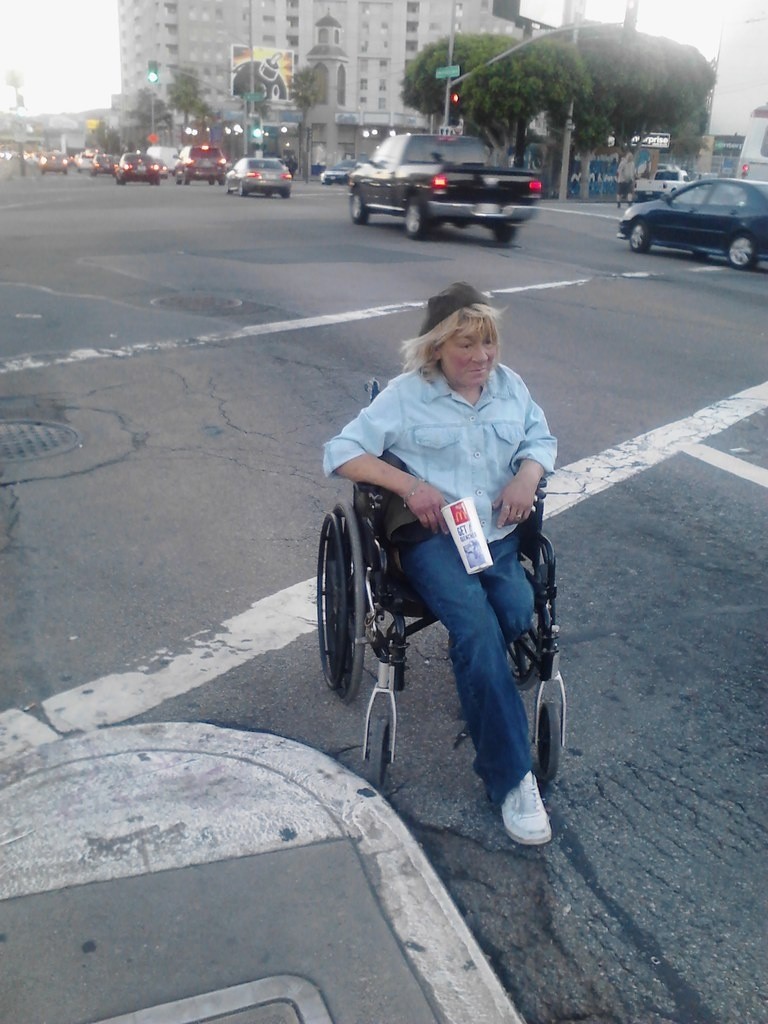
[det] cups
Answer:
[440,496,494,575]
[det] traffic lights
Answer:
[148,60,159,83]
[252,117,261,139]
[741,163,750,179]
[451,93,458,103]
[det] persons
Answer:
[615,152,637,208]
[321,282,558,847]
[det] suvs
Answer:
[172,146,226,186]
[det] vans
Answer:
[146,147,180,172]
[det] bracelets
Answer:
[404,477,426,508]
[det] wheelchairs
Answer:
[317,380,568,789]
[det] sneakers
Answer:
[500,769,551,845]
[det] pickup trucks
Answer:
[636,169,690,203]
[347,134,542,243]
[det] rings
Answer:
[502,504,510,510]
[516,514,522,518]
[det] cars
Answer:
[617,178,768,271]
[76,152,93,173]
[90,154,114,177]
[319,159,361,187]
[40,152,69,175]
[226,157,292,199]
[156,159,169,180]
[115,153,161,186]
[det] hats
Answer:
[419,280,489,338]
